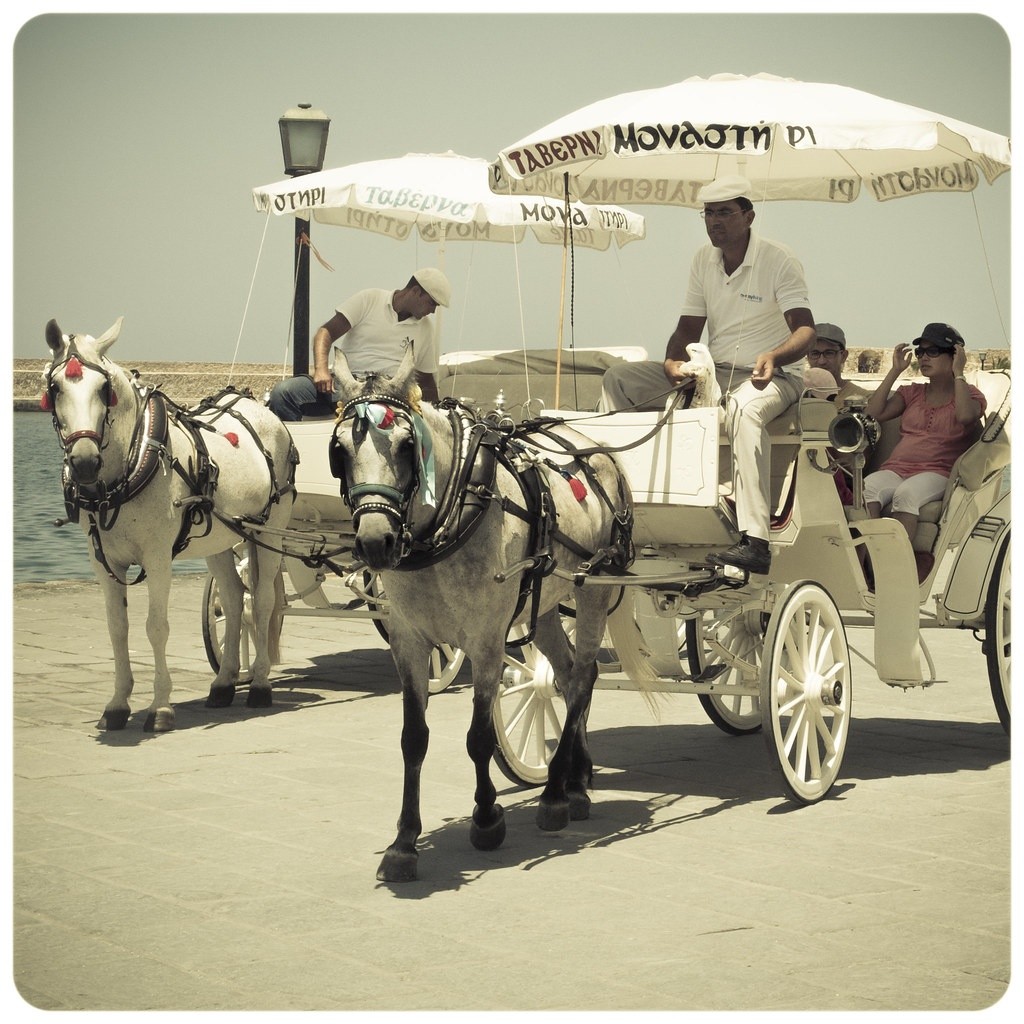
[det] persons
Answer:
[855,323,988,579]
[807,323,873,493]
[268,268,453,421]
[601,174,817,575]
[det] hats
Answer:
[913,323,965,352]
[698,174,751,202]
[814,323,846,349]
[413,268,450,308]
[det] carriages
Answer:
[38,315,648,738]
[325,360,1014,887]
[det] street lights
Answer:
[276,100,333,379]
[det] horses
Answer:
[329,339,634,884]
[40,313,301,733]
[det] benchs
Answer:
[440,372,602,422]
[847,388,1012,554]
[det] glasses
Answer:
[700,209,745,219]
[810,349,842,359]
[915,347,953,358]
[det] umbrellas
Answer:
[488,72,1012,208]
[252,151,645,251]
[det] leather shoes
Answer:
[706,534,772,576]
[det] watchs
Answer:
[954,376,966,382]
